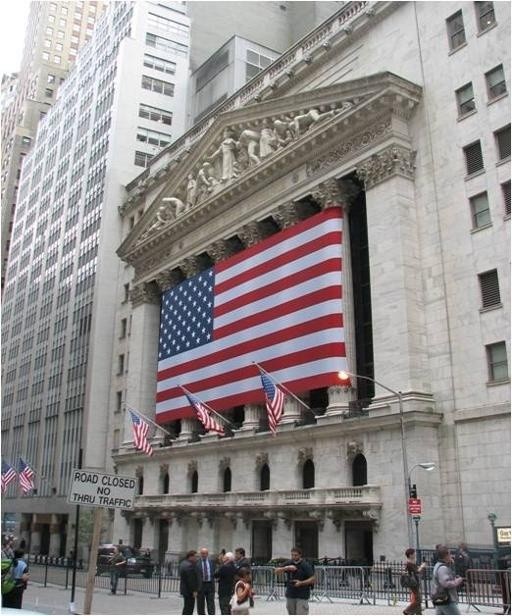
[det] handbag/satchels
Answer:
[401,573,418,587]
[233,594,250,610]
[433,590,450,605]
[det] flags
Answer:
[255,363,287,436]
[151,205,350,422]
[16,457,37,497]
[126,409,155,459]
[181,388,225,437]
[1,457,16,498]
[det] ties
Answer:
[203,559,208,580]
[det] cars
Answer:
[208,553,350,587]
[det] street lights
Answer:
[411,514,425,567]
[336,367,439,564]
[487,511,504,594]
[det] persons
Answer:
[143,546,153,558]
[273,545,317,614]
[106,544,128,597]
[1,531,31,609]
[398,538,475,615]
[177,547,255,615]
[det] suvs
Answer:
[93,542,155,579]
